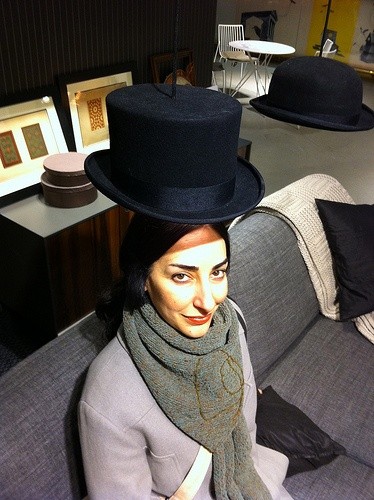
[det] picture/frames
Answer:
[66,69,133,154]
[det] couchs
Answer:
[0,173,374,500]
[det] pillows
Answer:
[317,197,372,320]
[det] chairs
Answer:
[212,25,258,95]
[314,39,337,58]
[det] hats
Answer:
[84,83,266,224]
[249,55,373,134]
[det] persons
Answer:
[76,213,292,500]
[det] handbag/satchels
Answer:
[257,385,346,477]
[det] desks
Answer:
[229,40,294,95]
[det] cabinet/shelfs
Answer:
[0,139,251,331]
